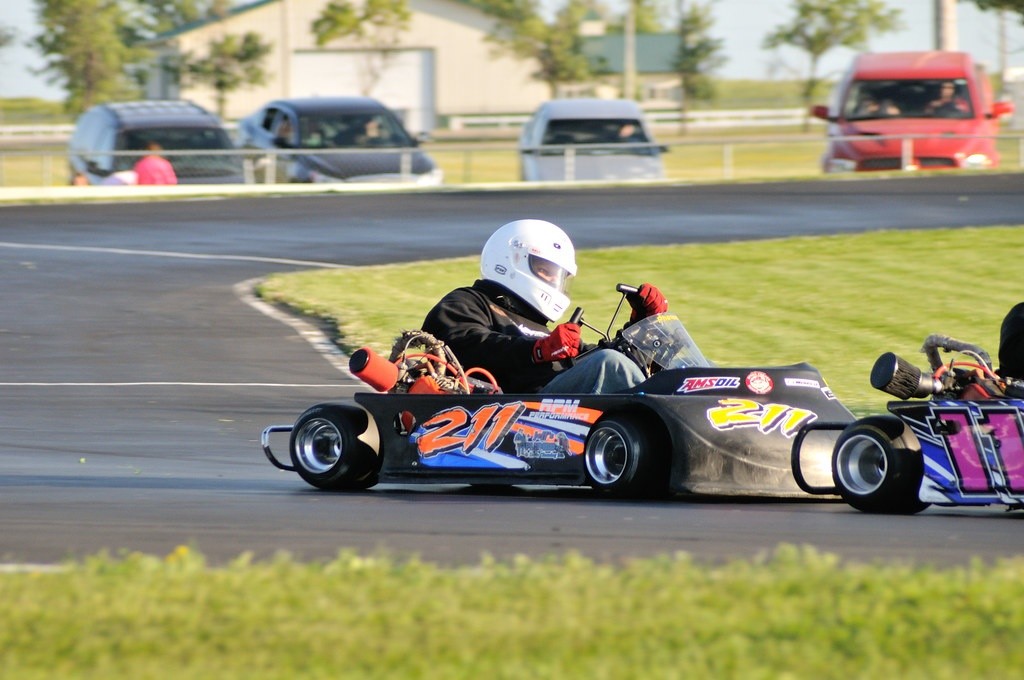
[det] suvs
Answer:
[66,97,244,187]
[807,48,1017,174]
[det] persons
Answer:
[421,220,668,395]
[616,125,636,140]
[924,80,970,115]
[273,114,321,145]
[998,302,1024,379]
[853,85,900,118]
[354,119,392,146]
[71,143,177,187]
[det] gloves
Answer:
[533,323,581,363]
[630,283,669,323]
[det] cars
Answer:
[515,98,672,183]
[233,97,444,189]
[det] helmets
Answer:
[479,219,576,322]
